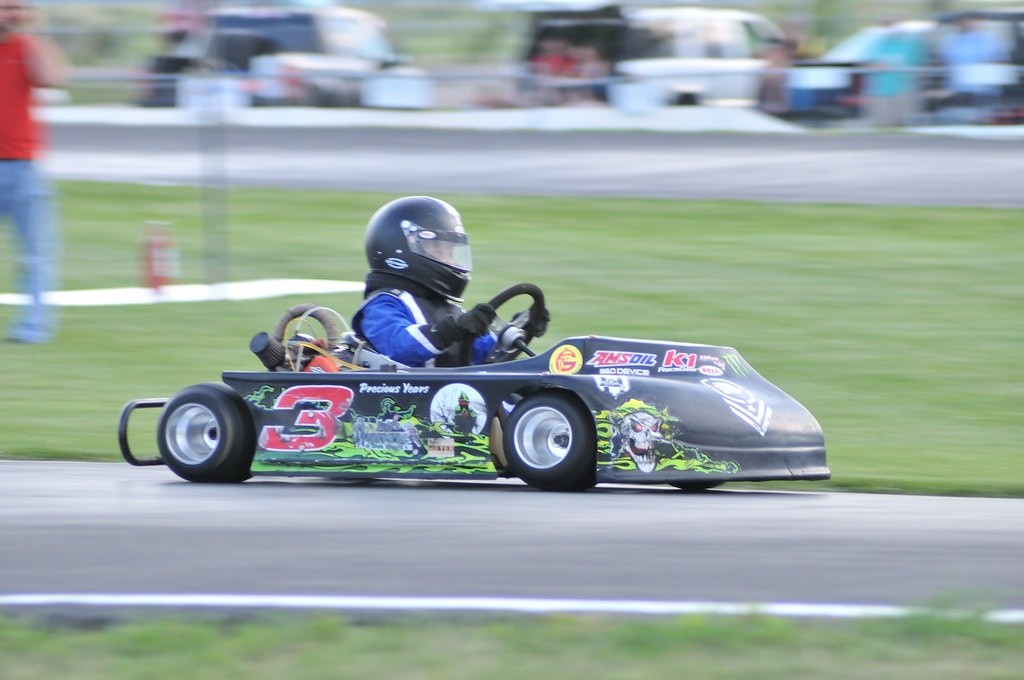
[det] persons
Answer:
[938,12,1001,124]
[0,0,73,344]
[143,13,202,97]
[531,32,616,106]
[351,196,550,368]
[861,8,925,123]
[364,60,434,106]
[758,38,813,108]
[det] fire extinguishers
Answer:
[140,219,179,295]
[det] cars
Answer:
[138,0,418,108]
[750,8,1024,124]
[472,1,802,112]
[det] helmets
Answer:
[364,196,471,303]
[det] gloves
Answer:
[513,303,551,338]
[455,303,497,338]
